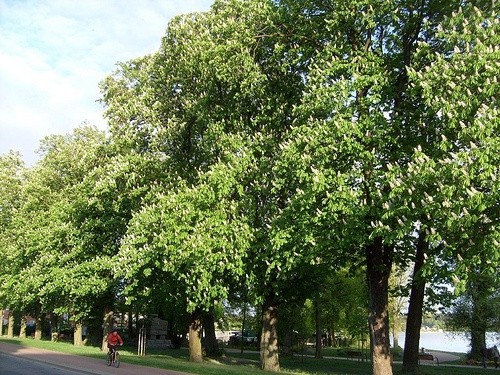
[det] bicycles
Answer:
[106,345,120,368]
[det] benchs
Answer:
[417,354,440,367]
[59,334,68,342]
[347,351,367,362]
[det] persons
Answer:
[107,329,123,364]
[422,347,430,355]
[323,335,332,347]
[492,345,499,368]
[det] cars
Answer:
[228,330,258,345]
